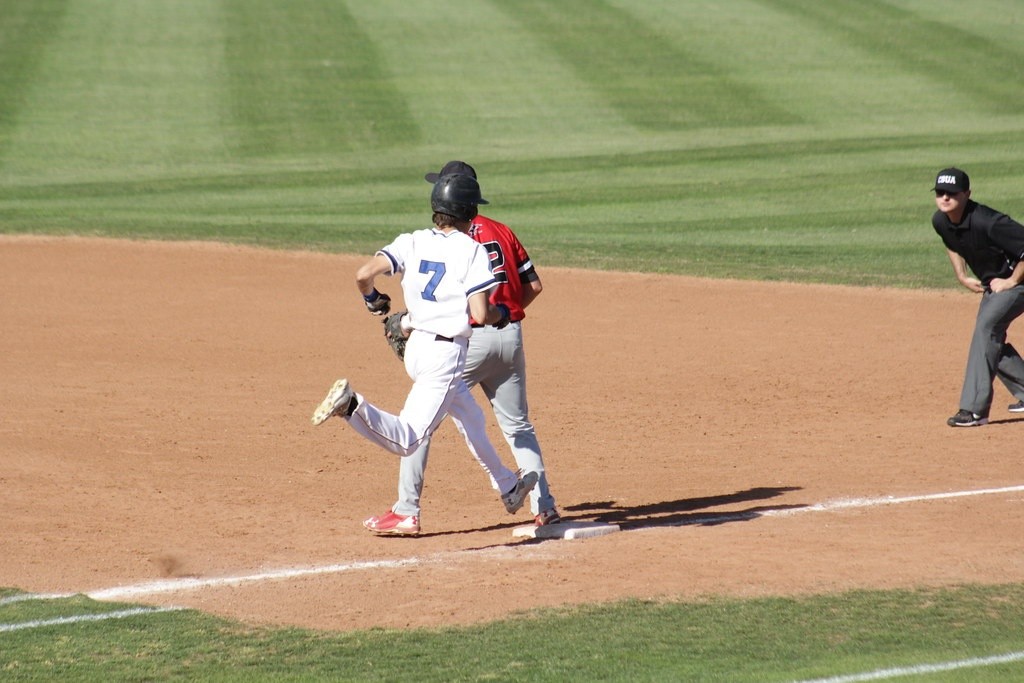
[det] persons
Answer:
[364,160,562,536]
[931,167,1024,427]
[312,174,538,512]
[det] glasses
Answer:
[936,190,957,196]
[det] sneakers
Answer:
[535,507,560,526]
[362,506,421,537]
[947,409,989,427]
[1008,400,1024,413]
[500,467,539,515]
[311,379,356,425]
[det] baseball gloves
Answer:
[382,311,410,363]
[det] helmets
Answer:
[431,173,490,223]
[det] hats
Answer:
[930,167,970,194]
[425,161,477,185]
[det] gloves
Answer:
[492,303,511,329]
[363,287,391,316]
